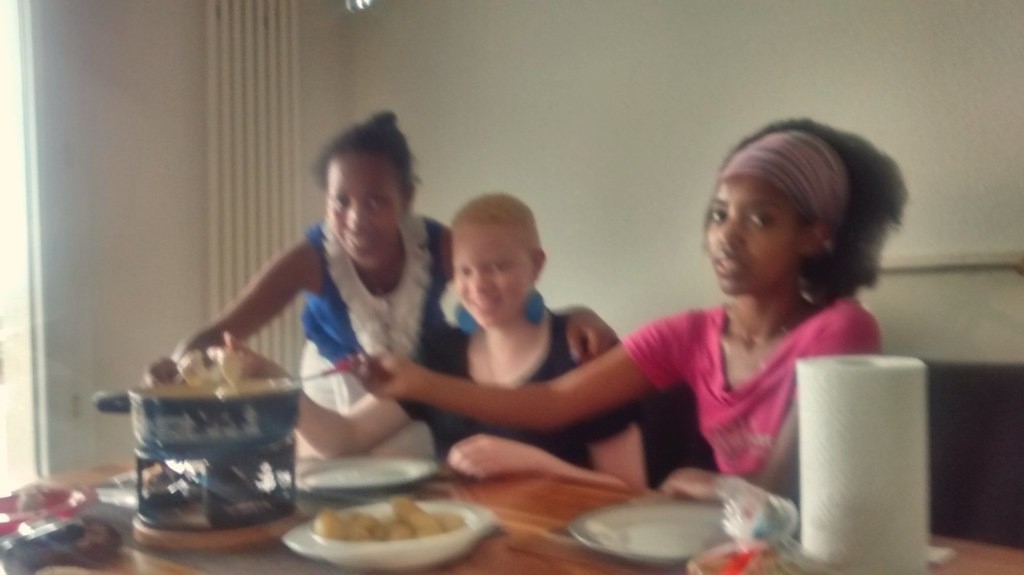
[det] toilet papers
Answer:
[799,354,932,570]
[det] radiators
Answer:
[205,0,300,384]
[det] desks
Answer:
[0,454,1024,575]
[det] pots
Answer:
[92,375,304,446]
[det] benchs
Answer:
[926,360,1024,550]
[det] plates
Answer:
[296,455,439,496]
[96,472,137,508]
[565,493,797,566]
[281,500,500,573]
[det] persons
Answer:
[223,192,646,493]
[144,111,619,412]
[343,118,906,501]
